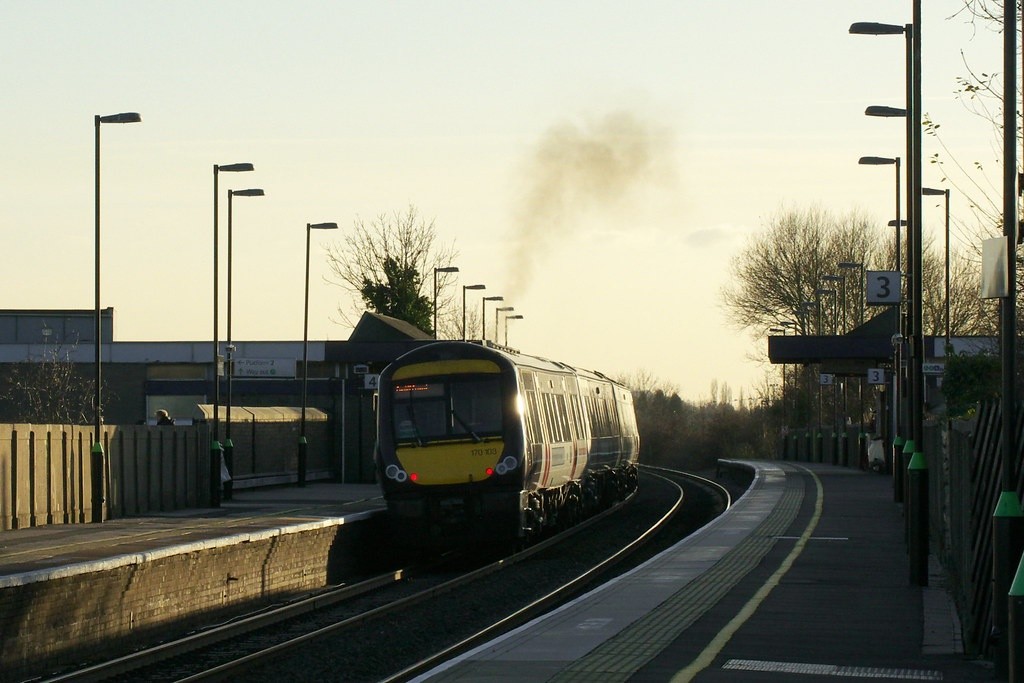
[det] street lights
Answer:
[463,285,486,342]
[802,301,825,464]
[482,296,504,340]
[211,162,256,509]
[504,315,524,347]
[814,288,838,465]
[791,310,812,463]
[224,188,266,506]
[494,306,514,345]
[780,321,798,464]
[838,263,866,472]
[921,188,952,361]
[297,222,339,487]
[768,328,789,461]
[851,22,918,556]
[859,155,907,505]
[90,111,142,524]
[434,266,460,340]
[822,274,849,468]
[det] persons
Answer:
[155,410,174,425]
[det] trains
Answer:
[370,336,641,552]
